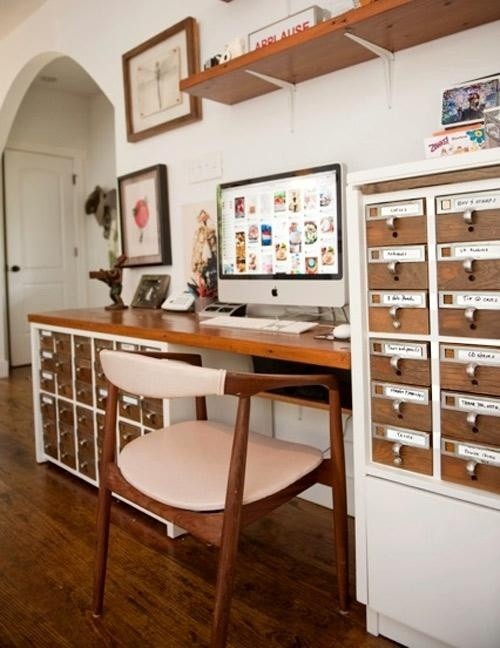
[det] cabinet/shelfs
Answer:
[345,149,499,646]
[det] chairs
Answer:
[92,347,351,645]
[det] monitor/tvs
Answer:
[216,161,349,321]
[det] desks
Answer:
[24,306,352,541]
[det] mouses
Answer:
[333,323,350,340]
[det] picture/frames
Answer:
[116,164,172,268]
[122,14,199,144]
[131,273,170,310]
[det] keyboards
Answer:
[201,315,320,334]
